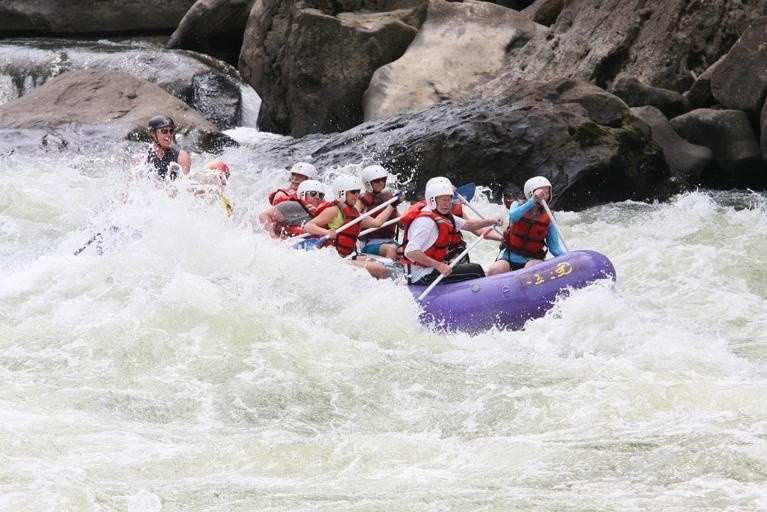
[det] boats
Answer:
[96,205,617,335]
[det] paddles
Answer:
[358,183,475,237]
[289,191,402,251]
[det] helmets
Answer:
[297,180,325,197]
[524,176,552,206]
[149,115,175,132]
[425,177,454,210]
[291,162,316,180]
[360,165,388,193]
[333,175,361,204]
[209,162,229,177]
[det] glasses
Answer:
[350,190,360,195]
[307,191,324,199]
[374,178,385,183]
[161,128,174,134]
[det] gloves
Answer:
[390,192,403,208]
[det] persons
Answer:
[191,161,234,216]
[129,112,191,198]
[486,174,566,278]
[258,161,504,286]
